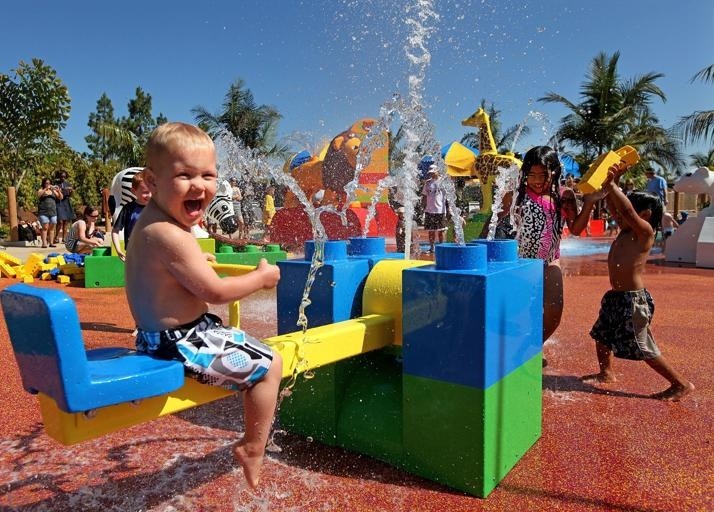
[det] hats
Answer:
[426,164,440,175]
[646,168,655,173]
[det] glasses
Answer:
[89,215,99,218]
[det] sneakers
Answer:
[425,248,435,254]
[41,238,66,247]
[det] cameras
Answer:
[67,187,73,190]
[50,185,57,190]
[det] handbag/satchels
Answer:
[18,223,37,241]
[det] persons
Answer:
[577,162,696,402]
[124,122,283,487]
[479,146,606,367]
[0,169,105,256]
[112,169,154,262]
[229,176,276,239]
[397,165,469,253]
[560,167,689,248]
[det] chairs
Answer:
[0,283,186,410]
